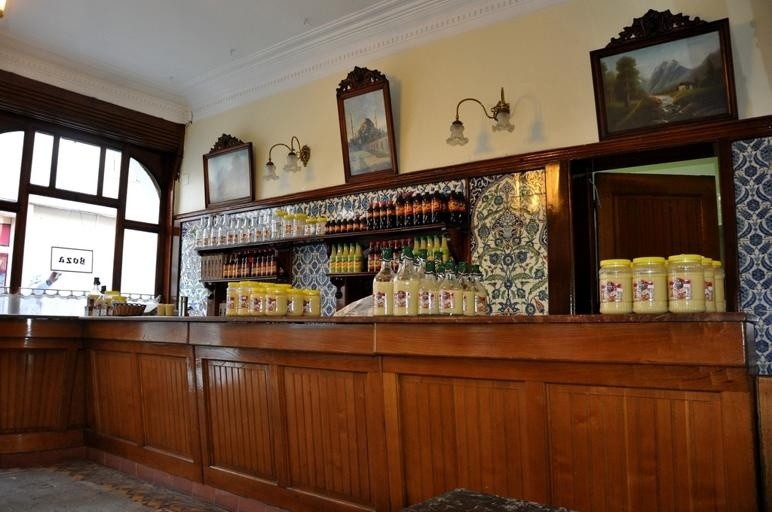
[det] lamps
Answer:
[262,135,312,183]
[447,87,516,148]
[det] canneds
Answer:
[225,280,320,316]
[598,254,726,315]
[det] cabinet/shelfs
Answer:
[194,240,293,284]
[323,222,473,278]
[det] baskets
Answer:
[113,304,146,316]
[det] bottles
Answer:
[180,296,188,316]
[194,206,328,244]
[84,276,127,316]
[323,190,490,319]
[223,246,283,280]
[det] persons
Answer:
[23,272,60,296]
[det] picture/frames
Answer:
[585,8,739,144]
[333,64,400,183]
[200,131,257,209]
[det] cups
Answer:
[156,304,174,316]
